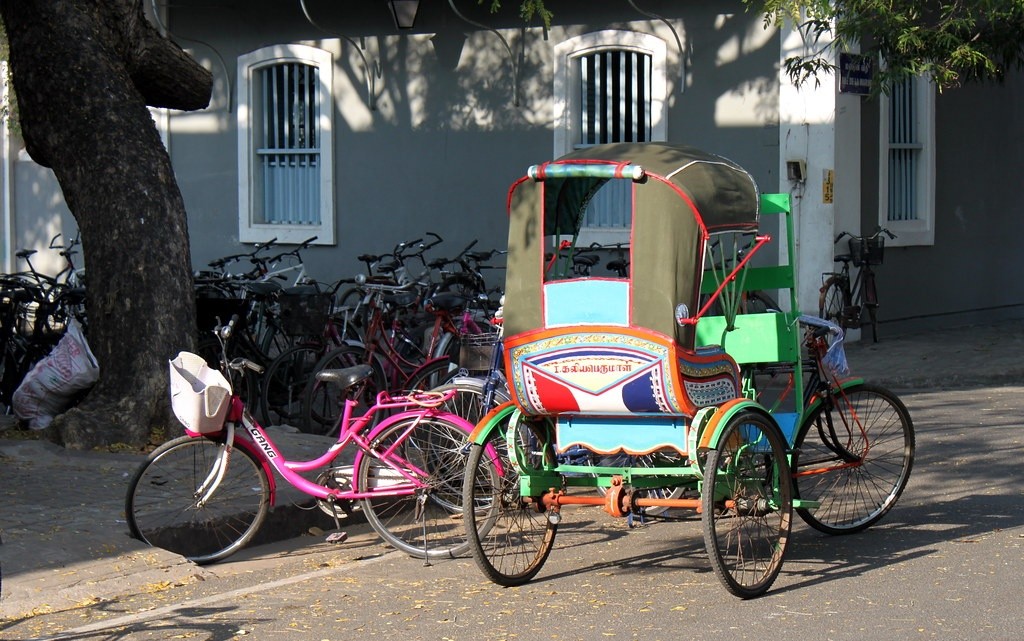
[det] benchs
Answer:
[503,277,743,416]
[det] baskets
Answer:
[168,351,233,434]
[848,236,885,267]
[460,331,499,370]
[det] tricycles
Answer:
[457,142,918,599]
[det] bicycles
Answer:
[1,215,631,515]
[123,310,504,566]
[819,225,898,343]
